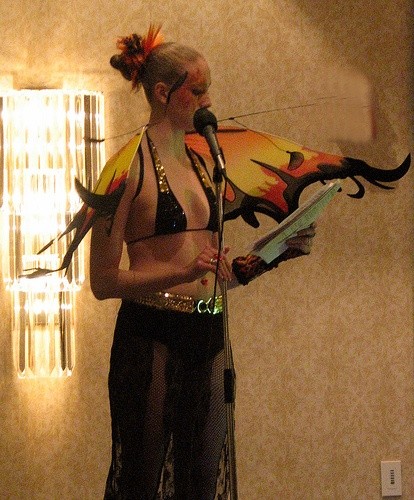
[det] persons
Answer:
[88,34,316,500]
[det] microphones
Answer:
[193,109,227,175]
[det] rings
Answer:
[308,237,311,246]
[210,258,217,264]
